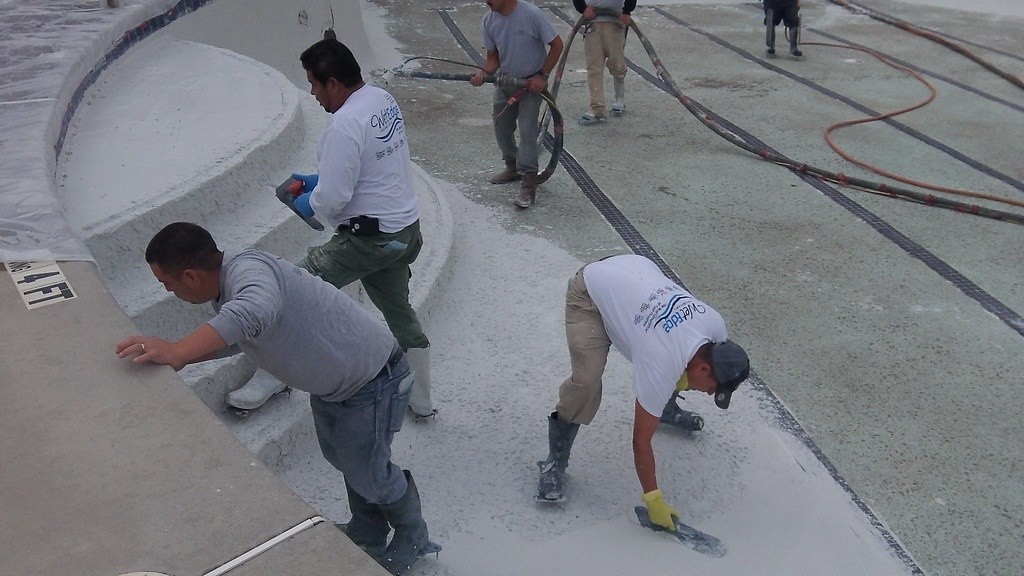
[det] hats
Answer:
[711,338,751,410]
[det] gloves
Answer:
[291,172,319,219]
[676,370,688,391]
[641,487,681,531]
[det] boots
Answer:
[659,389,705,431]
[513,172,538,208]
[332,475,392,554]
[767,25,775,54]
[583,110,607,122]
[537,411,580,501]
[403,343,434,417]
[612,76,626,113]
[491,160,521,183]
[226,367,287,410]
[378,468,429,576]
[789,26,803,56]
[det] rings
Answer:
[141,343,144,354]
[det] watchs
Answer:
[539,70,549,80]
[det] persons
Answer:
[537,254,751,530]
[116,222,430,576]
[469,0,565,208]
[226,38,439,417]
[572,0,637,120]
[761,0,803,56]
